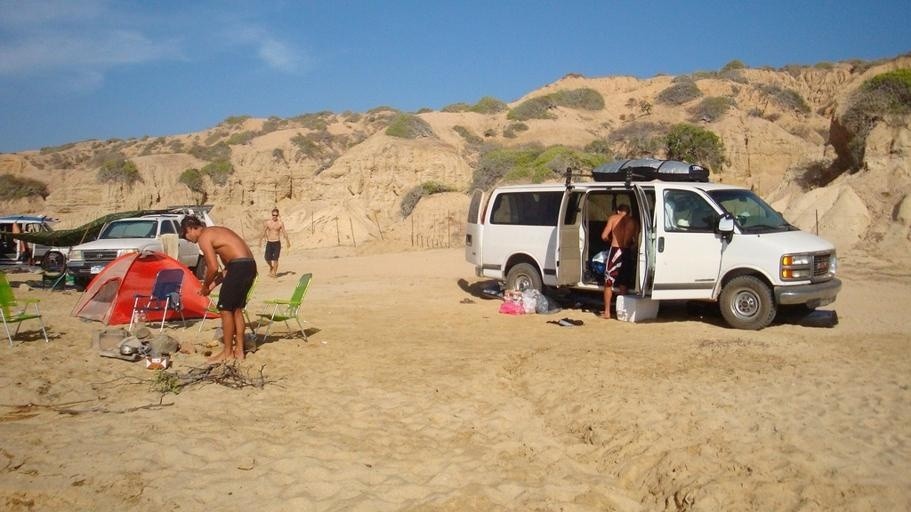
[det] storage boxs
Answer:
[614,294,660,322]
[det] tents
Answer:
[71,248,220,326]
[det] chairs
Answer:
[0,272,49,347]
[197,272,259,339]
[254,272,314,345]
[125,267,189,335]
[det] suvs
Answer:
[0,215,62,268]
[67,213,205,292]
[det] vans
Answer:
[466,168,843,332]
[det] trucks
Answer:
[29,203,214,273]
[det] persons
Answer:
[178,216,258,362]
[258,207,291,272]
[599,204,639,322]
